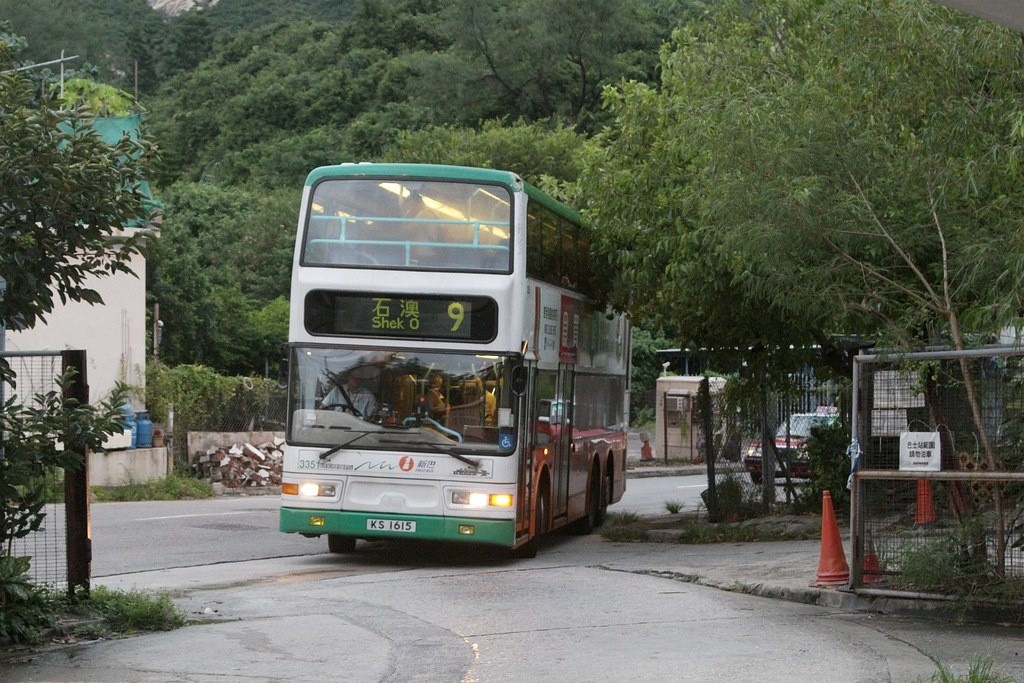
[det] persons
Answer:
[373,355,497,437]
[402,194,443,267]
[316,367,382,423]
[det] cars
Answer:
[743,404,843,485]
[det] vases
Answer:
[153,435,163,447]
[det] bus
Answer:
[279,157,633,557]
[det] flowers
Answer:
[153,430,161,436]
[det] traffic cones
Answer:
[914,477,941,523]
[862,527,887,583]
[810,488,850,587]
